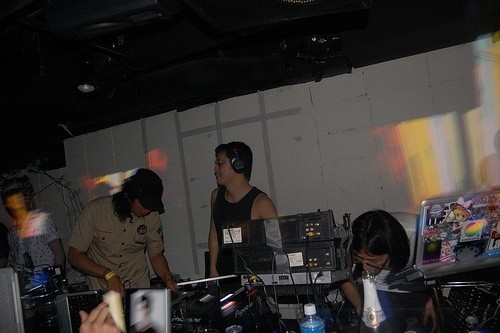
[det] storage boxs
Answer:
[278,303,304,320]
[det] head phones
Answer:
[230,144,246,173]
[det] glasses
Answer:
[351,251,391,271]
[215,160,230,165]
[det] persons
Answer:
[208,141,278,278]
[0,179,65,289]
[68,168,181,296]
[340,209,451,333]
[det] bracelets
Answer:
[103,268,117,281]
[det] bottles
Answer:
[299,303,325,333]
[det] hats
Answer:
[131,168,164,210]
[0,177,29,195]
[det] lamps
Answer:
[75,56,100,94]
